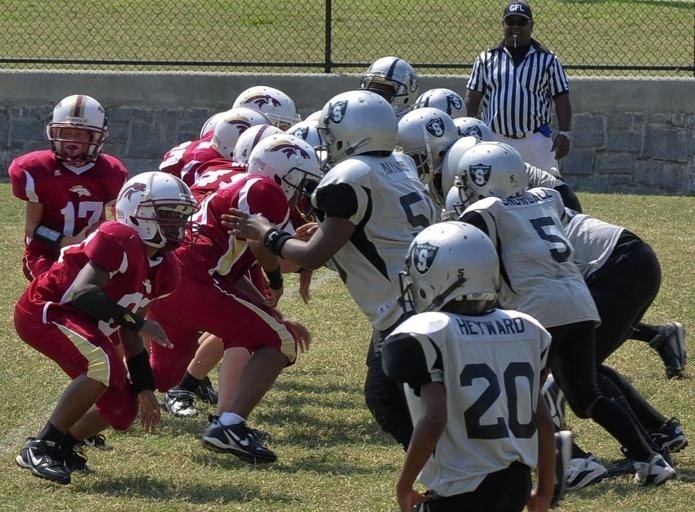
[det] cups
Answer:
[505,16,528,26]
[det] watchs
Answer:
[559,130,571,139]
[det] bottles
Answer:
[503,2,532,20]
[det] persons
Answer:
[463,3,571,180]
[379,221,556,510]
[7,58,689,509]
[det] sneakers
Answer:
[649,321,687,378]
[160,388,201,417]
[202,413,276,463]
[15,437,71,485]
[83,433,106,447]
[550,430,608,507]
[196,378,218,405]
[609,446,676,486]
[63,443,88,473]
[649,416,688,453]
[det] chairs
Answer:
[15,437,71,485]
[649,321,687,378]
[550,430,608,507]
[202,413,276,463]
[196,378,218,405]
[160,388,201,417]
[649,416,688,453]
[63,443,88,473]
[609,446,676,486]
[83,433,106,447]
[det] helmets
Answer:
[201,112,225,136]
[453,117,493,142]
[458,141,526,205]
[232,86,297,129]
[306,112,323,120]
[322,91,397,166]
[397,107,460,173]
[248,133,324,201]
[233,125,286,163]
[46,94,108,166]
[285,120,327,153]
[414,89,467,119]
[211,107,273,161]
[116,171,201,251]
[357,56,419,115]
[397,221,501,315]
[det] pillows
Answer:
[116,171,201,251]
[357,56,419,115]
[397,221,501,315]
[46,94,108,166]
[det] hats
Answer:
[503,2,532,20]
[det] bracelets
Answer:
[265,227,296,260]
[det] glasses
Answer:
[505,16,528,26]
